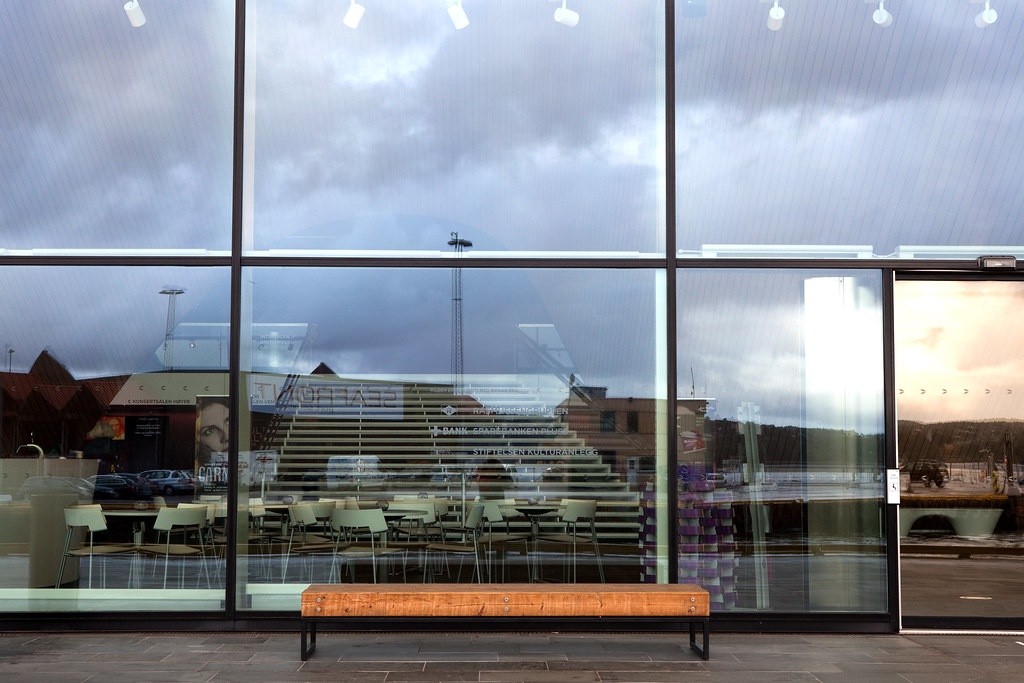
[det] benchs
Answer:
[300,583,711,662]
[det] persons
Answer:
[87,417,125,440]
[196,397,230,495]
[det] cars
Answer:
[688,472,729,493]
[15,468,203,508]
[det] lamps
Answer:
[554,0,580,27]
[343,0,365,28]
[767,0,785,31]
[448,0,470,30]
[873,0,892,26]
[975,0,997,28]
[124,0,146,27]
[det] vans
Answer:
[326,455,394,492]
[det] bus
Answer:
[502,464,554,482]
[430,464,479,482]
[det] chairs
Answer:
[56,495,605,589]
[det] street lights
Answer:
[8,348,14,373]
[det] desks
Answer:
[380,510,428,584]
[101,510,159,588]
[498,505,568,583]
[263,504,290,582]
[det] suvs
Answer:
[899,458,951,489]
